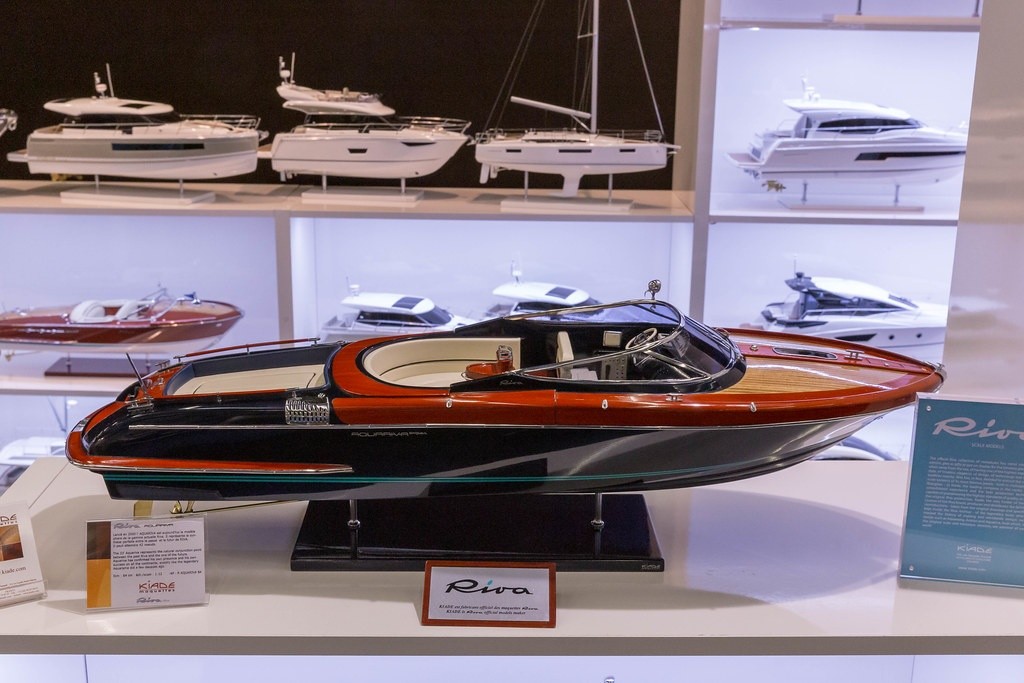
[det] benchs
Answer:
[557,331,598,380]
[73,299,104,324]
[116,301,142,320]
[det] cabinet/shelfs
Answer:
[0,0,979,459]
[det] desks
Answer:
[0,461,1024,656]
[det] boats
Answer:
[6,65,270,180]
[256,53,473,178]
[758,268,947,360]
[726,78,969,187]
[63,280,947,495]
[314,283,652,343]
[0,435,67,495]
[0,287,246,353]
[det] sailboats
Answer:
[479,0,679,172]
